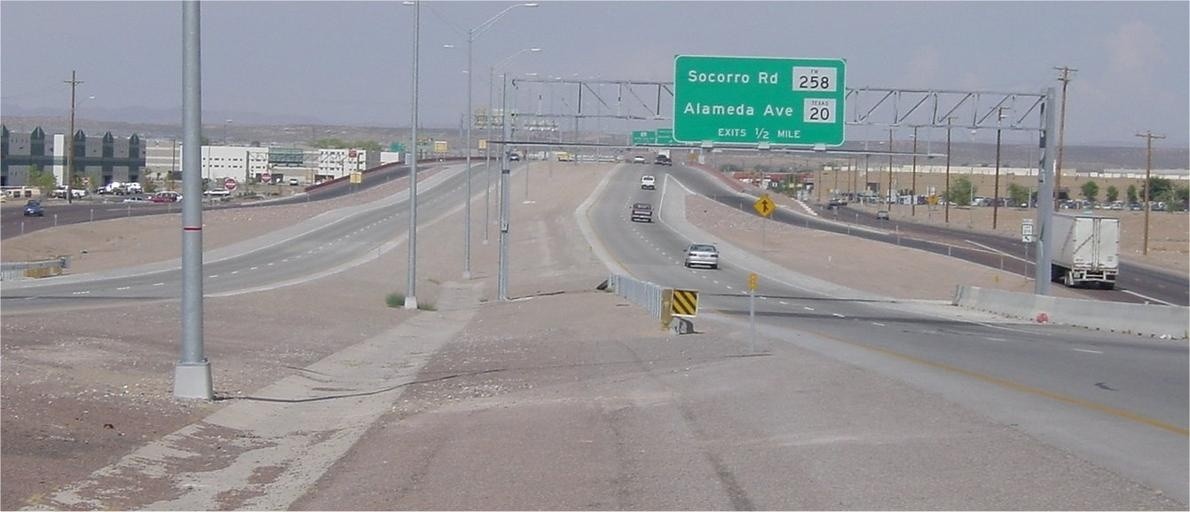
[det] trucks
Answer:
[1047,212,1120,290]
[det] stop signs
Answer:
[224,176,238,191]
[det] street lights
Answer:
[66,96,96,204]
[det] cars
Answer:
[96,181,182,204]
[877,210,889,221]
[262,173,298,186]
[24,200,44,216]
[204,187,230,198]
[1055,197,1189,212]
[683,242,719,269]
[830,198,847,206]
[631,149,671,190]
[973,196,1039,208]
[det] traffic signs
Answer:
[672,55,848,151]
[631,128,672,146]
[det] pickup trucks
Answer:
[628,202,655,223]
[51,185,86,199]
